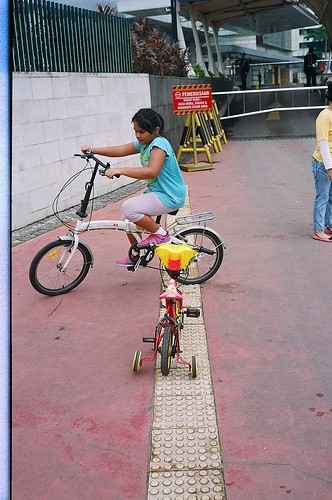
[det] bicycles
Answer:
[27,149,227,297]
[131,245,198,380]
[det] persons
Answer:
[81,108,186,266]
[311,80,332,242]
[237,52,249,90]
[258,71,262,86]
[304,46,316,86]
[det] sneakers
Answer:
[137,230,172,246]
[116,256,147,267]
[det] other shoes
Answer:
[313,232,332,242]
[325,228,332,233]
